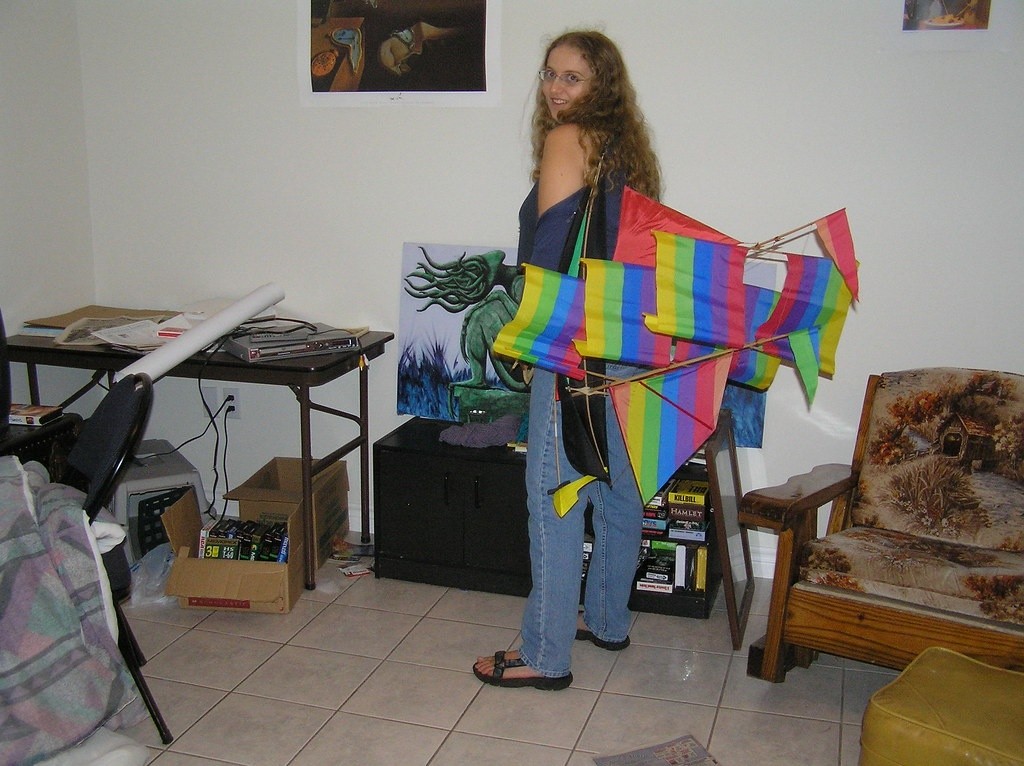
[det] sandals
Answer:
[472,651,572,690]
[575,610,631,651]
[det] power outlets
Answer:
[223,388,241,419]
[7,304,396,590]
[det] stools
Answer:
[856,647,1024,766]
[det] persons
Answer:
[472,31,663,690]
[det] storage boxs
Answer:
[221,456,351,570]
[159,489,302,615]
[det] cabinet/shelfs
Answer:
[373,416,723,620]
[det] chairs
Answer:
[53,373,173,745]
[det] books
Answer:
[9,404,64,426]
[158,324,189,339]
[198,519,289,563]
[581,479,709,593]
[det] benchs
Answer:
[737,366,1024,683]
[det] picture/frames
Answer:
[705,409,756,651]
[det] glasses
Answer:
[539,68,591,87]
